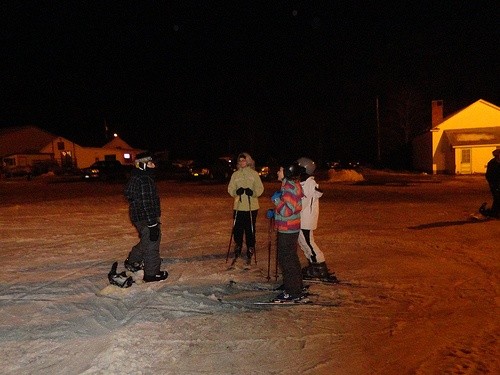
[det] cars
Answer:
[25,159,55,181]
[185,159,272,181]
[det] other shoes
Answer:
[276,290,301,301]
[279,284,285,291]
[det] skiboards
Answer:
[216,279,342,307]
[275,269,347,287]
[226,248,256,271]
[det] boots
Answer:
[247,247,254,264]
[235,241,242,257]
[302,262,327,280]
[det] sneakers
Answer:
[143,271,167,281]
[124,258,145,272]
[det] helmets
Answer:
[296,158,315,176]
[281,161,299,179]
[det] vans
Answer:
[84,160,121,181]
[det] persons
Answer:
[485,149,500,219]
[266,161,304,303]
[297,158,329,278]
[227,154,264,258]
[124,153,168,282]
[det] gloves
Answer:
[244,188,253,197]
[236,188,244,195]
[147,222,159,242]
[272,192,281,201]
[266,209,274,219]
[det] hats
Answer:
[134,153,153,163]
